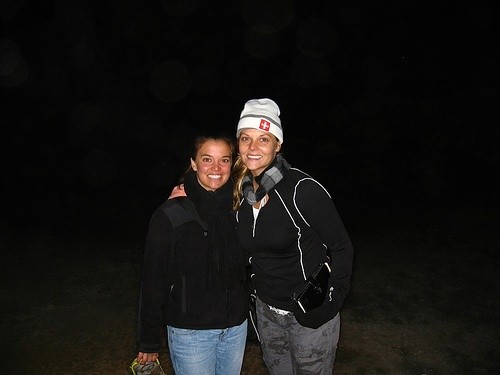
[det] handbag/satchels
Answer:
[294,259,331,313]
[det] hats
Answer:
[237,99,283,145]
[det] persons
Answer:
[167,97,354,375]
[136,129,254,375]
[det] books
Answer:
[294,262,332,314]
